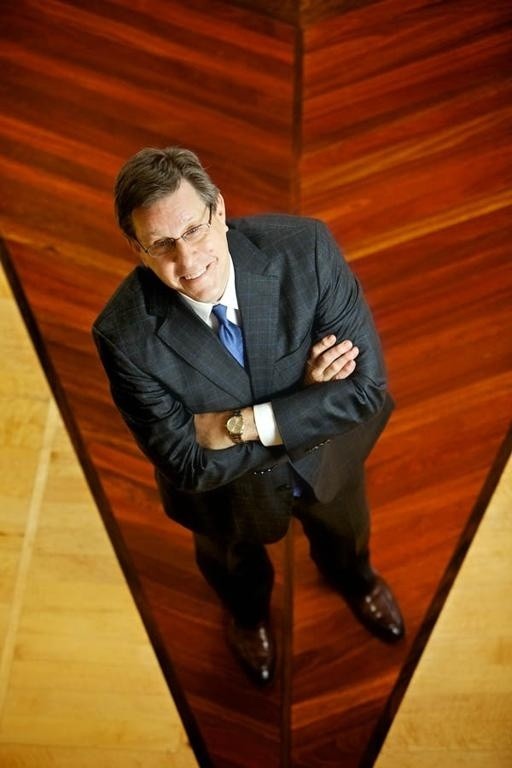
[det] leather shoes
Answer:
[227,618,276,690]
[353,576,404,644]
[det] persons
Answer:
[92,145,404,681]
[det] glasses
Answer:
[134,203,213,258]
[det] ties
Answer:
[212,304,248,370]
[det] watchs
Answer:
[225,408,247,446]
